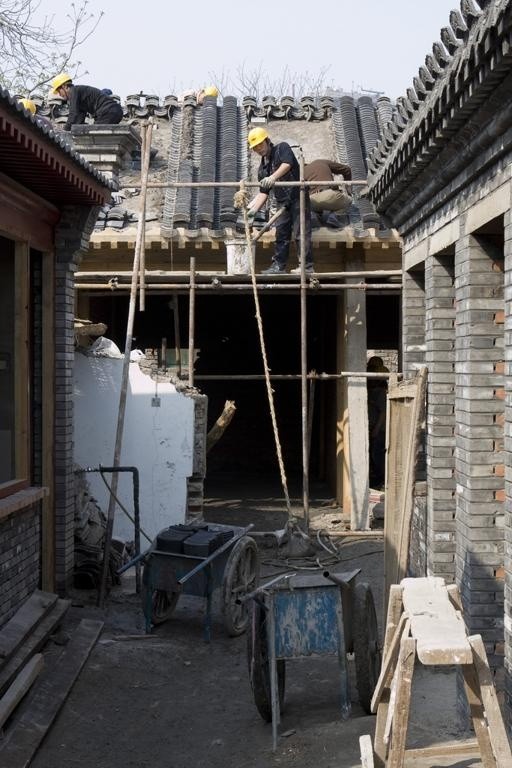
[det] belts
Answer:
[312,186,338,193]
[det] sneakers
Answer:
[327,216,345,228]
[290,265,314,273]
[260,263,287,275]
[311,218,323,227]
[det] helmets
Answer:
[247,127,269,149]
[52,74,72,95]
[17,97,36,115]
[204,87,218,98]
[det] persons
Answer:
[51,74,124,131]
[364,354,391,448]
[178,87,219,105]
[299,158,354,228]
[241,126,317,274]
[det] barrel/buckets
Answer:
[224,239,256,275]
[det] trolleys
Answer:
[235,568,381,755]
[118,515,261,644]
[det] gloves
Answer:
[247,208,257,224]
[260,177,275,189]
